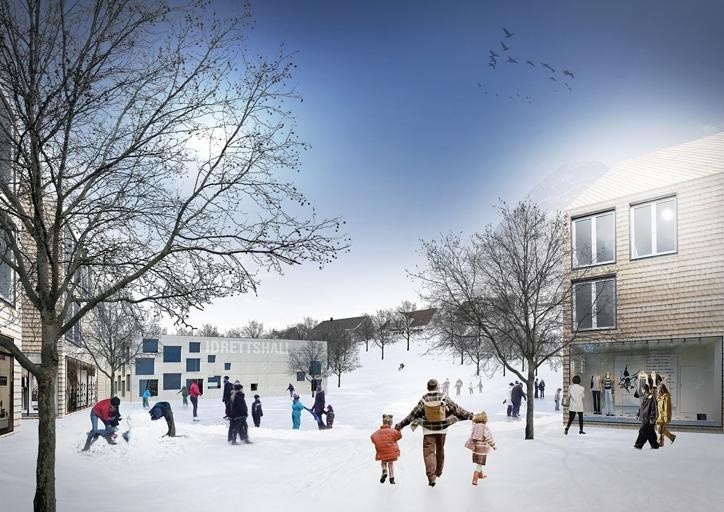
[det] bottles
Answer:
[383,414,393,426]
[234,385,243,390]
[254,395,259,399]
[294,394,299,399]
[427,379,439,391]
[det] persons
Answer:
[535,378,539,398]
[554,388,562,410]
[505,380,527,418]
[648,370,663,398]
[395,379,473,487]
[633,383,659,449]
[564,375,586,435]
[635,370,648,396]
[442,376,484,395]
[371,414,402,484]
[142,379,201,438]
[82,398,123,450]
[222,376,263,445]
[399,362,405,370]
[601,371,616,416]
[539,380,545,397]
[286,382,336,430]
[464,411,497,485]
[590,375,602,414]
[657,383,676,446]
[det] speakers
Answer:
[472,470,487,485]
[380,469,395,483]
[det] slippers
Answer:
[420,394,449,422]
